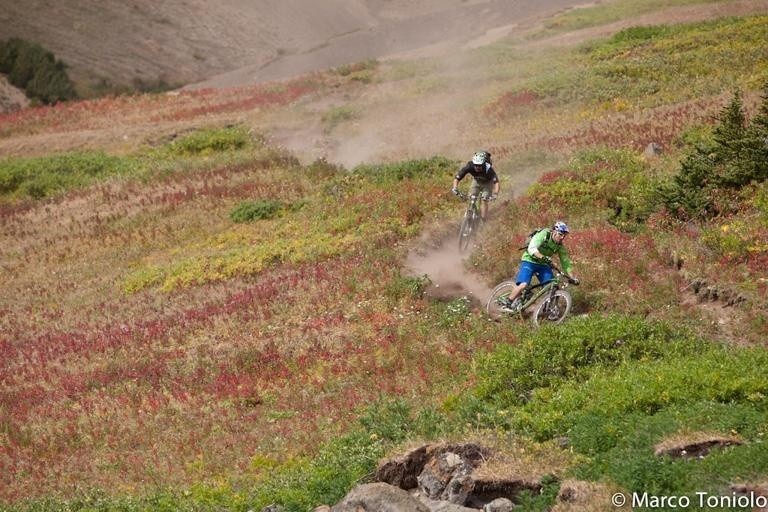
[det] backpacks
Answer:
[485,152,492,165]
[525,227,550,249]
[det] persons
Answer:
[453,150,500,232]
[496,221,580,315]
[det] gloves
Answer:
[489,194,497,201]
[570,277,580,285]
[452,188,459,195]
[542,256,552,263]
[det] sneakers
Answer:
[502,297,512,310]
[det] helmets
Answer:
[553,222,569,233]
[473,152,486,164]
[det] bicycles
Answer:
[485,257,580,330]
[454,188,495,254]
